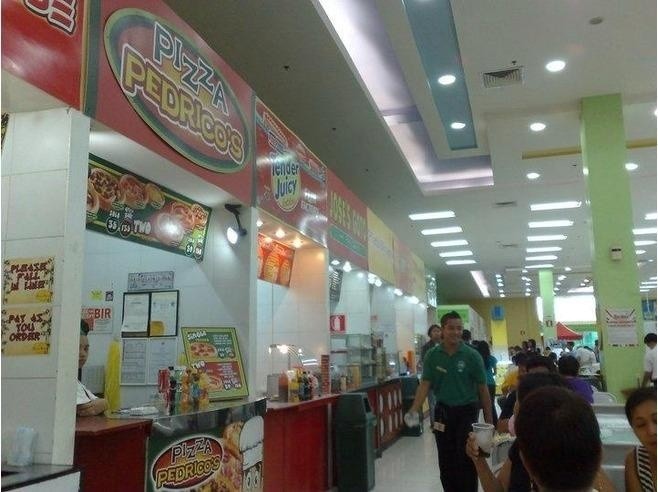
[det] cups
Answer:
[470,422,495,459]
[5,424,38,467]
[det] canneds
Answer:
[158,367,184,416]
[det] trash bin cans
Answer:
[401,376,424,437]
[332,392,376,492]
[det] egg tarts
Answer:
[204,376,223,393]
[86,167,208,248]
[190,341,217,359]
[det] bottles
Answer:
[166,365,210,414]
[276,368,323,406]
[330,365,353,394]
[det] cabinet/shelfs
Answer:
[332,332,374,379]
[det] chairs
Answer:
[492,365,641,491]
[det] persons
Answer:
[624,387,656,491]
[465,338,614,492]
[411,311,499,491]
[641,333,656,387]
[76,318,106,416]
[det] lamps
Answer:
[222,203,248,247]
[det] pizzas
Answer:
[223,420,245,448]
[196,438,244,492]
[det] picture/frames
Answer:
[122,292,151,337]
[149,289,180,337]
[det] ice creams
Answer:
[257,241,291,286]
